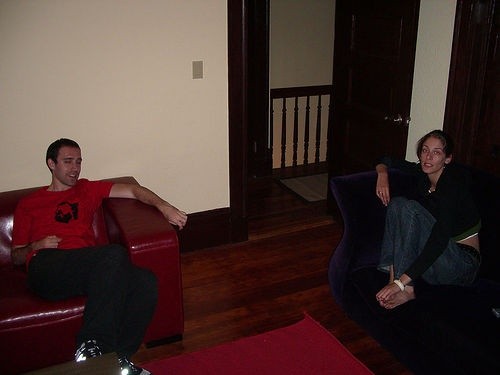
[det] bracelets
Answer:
[394,279,405,292]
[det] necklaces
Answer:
[428,188,436,194]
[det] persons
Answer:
[376,130,481,310]
[10,137,187,375]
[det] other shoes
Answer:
[75,340,100,363]
[117,356,152,375]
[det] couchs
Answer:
[0,177,184,375]
[329,168,500,375]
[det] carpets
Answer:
[137,312,372,375]
[274,172,328,205]
